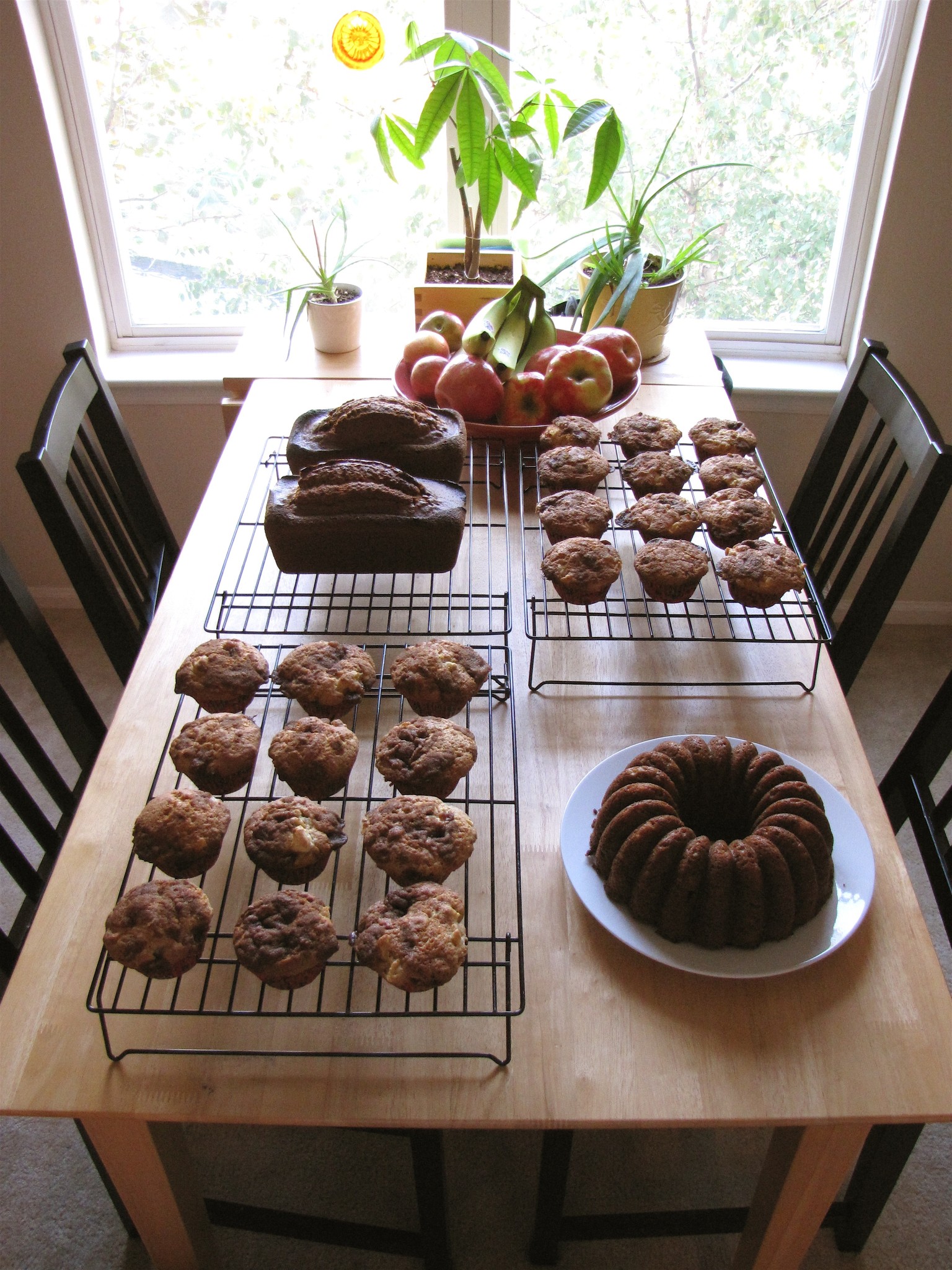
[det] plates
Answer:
[559,733,875,980]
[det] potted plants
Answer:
[268,207,398,360]
[370,20,627,328]
[528,98,758,364]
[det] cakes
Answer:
[587,734,833,951]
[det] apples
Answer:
[402,310,642,429]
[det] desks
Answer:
[1,378,952,1270]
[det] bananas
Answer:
[460,274,557,382]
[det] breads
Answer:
[285,396,466,482]
[376,715,479,805]
[536,413,807,611]
[132,788,232,880]
[390,640,492,719]
[263,458,466,574]
[169,712,261,795]
[233,888,339,990]
[270,641,376,721]
[362,793,476,887]
[175,637,269,713]
[243,795,348,885]
[102,878,214,980]
[268,715,359,800]
[348,881,467,992]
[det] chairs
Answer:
[783,337,952,695]
[14,337,182,684]
[0,553,454,1270]
[528,671,948,1270]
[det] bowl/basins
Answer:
[392,326,642,449]
